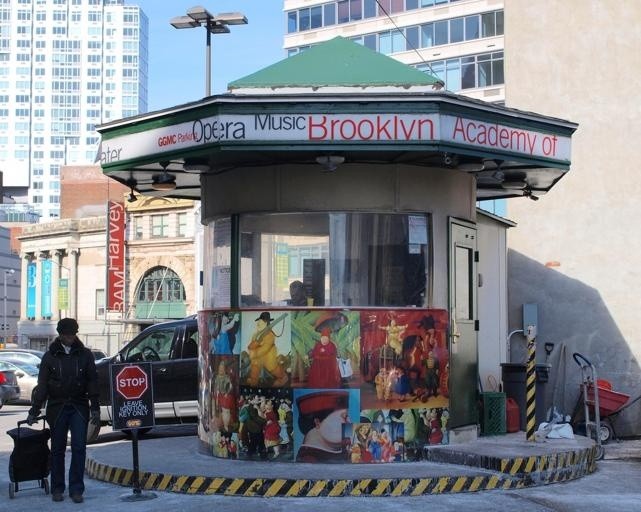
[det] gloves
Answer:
[90,411,100,425]
[248,341,259,350]
[27,415,38,426]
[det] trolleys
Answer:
[6,416,51,498]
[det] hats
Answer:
[255,312,274,321]
[296,391,349,415]
[321,328,329,337]
[57,318,78,332]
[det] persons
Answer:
[211,311,440,463]
[288,279,307,305]
[26,317,101,504]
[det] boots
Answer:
[270,365,288,387]
[247,365,260,385]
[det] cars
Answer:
[0,348,108,409]
[96,319,199,403]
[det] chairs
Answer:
[177,336,198,360]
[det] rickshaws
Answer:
[573,352,630,439]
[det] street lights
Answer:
[169,6,248,98]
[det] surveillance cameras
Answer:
[530,196,539,201]
[443,156,452,165]
[491,171,505,181]
[127,196,137,203]
[321,160,337,175]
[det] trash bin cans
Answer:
[500,362,552,432]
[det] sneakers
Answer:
[73,494,83,503]
[53,493,64,501]
